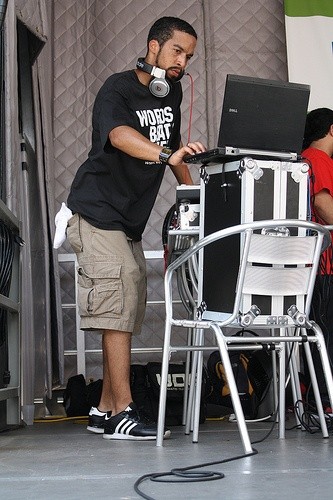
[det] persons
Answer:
[51,15,199,441]
[296,107,333,420]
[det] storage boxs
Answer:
[195,158,310,323]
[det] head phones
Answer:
[136,57,189,98]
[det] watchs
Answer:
[159,147,174,164]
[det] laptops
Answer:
[183,74,311,163]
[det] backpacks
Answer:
[207,330,263,419]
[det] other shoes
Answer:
[309,413,333,429]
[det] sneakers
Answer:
[103,402,170,440]
[87,403,155,433]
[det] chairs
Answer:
[156,219,333,453]
